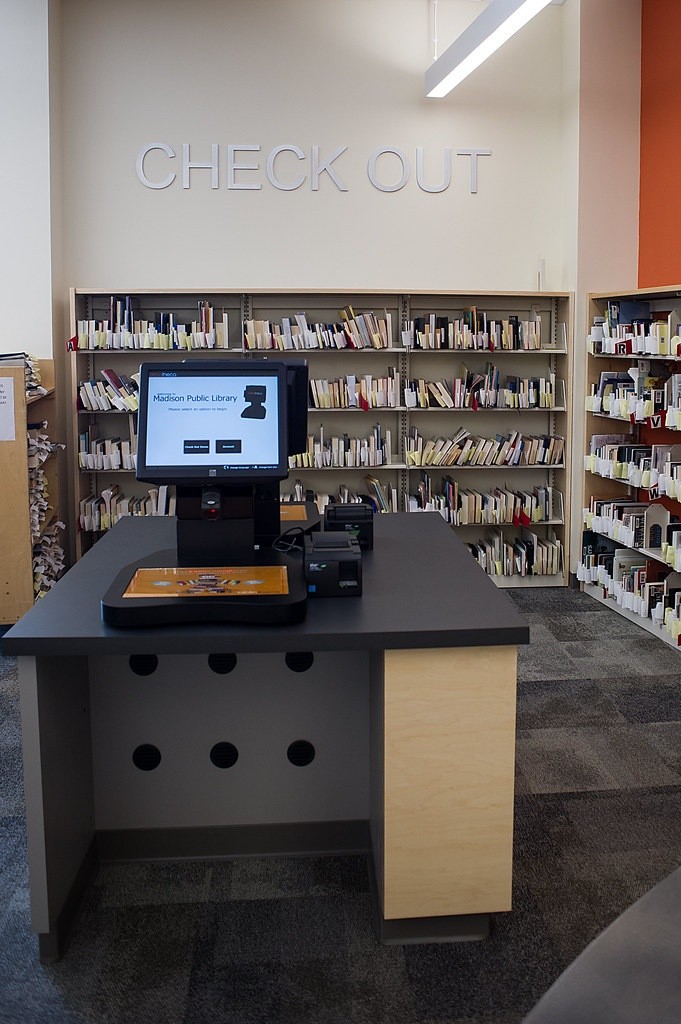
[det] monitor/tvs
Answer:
[135,360,308,485]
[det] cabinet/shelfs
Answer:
[0,285,681,648]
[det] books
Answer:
[288,424,392,468]
[0,352,48,400]
[409,471,553,524]
[79,413,138,470]
[580,433,681,571]
[26,421,66,469]
[402,425,565,466]
[310,366,402,409]
[410,305,542,351]
[33,516,67,603]
[465,527,562,576]
[244,303,392,351]
[29,470,54,543]
[576,530,681,647]
[65,296,228,352]
[408,361,556,408]
[78,485,176,532]
[279,474,398,514]
[586,300,681,431]
[77,369,140,412]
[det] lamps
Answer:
[423,0,552,99]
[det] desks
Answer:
[0,510,529,966]
